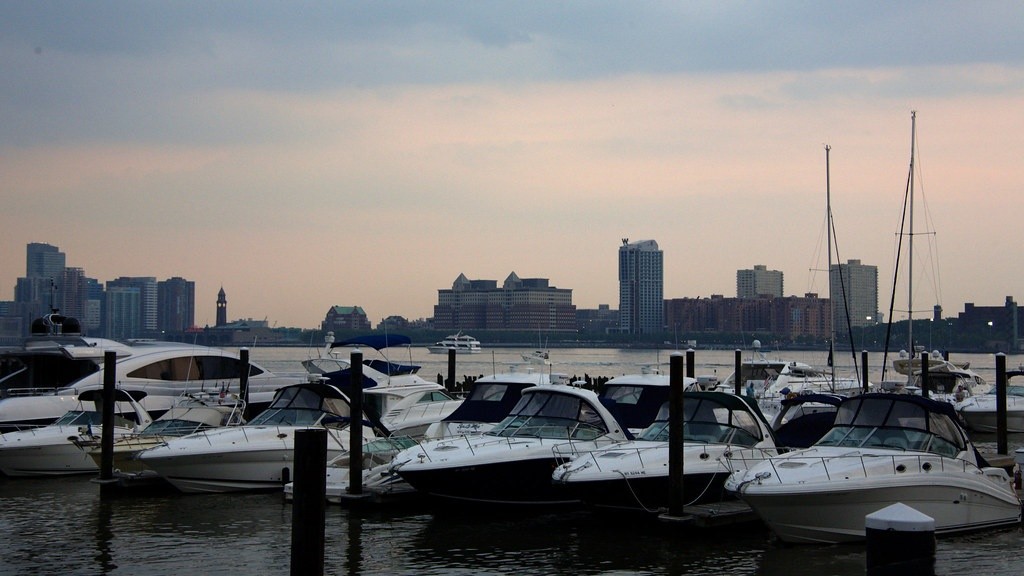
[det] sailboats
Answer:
[783,110,990,448]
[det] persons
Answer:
[746,382,755,398]
[955,385,965,403]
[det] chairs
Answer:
[883,436,908,449]
[859,434,881,445]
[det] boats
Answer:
[722,343,1024,548]
[0,275,463,504]
[380,324,818,531]
[520,326,551,365]
[955,354,1024,434]
[427,329,482,354]
